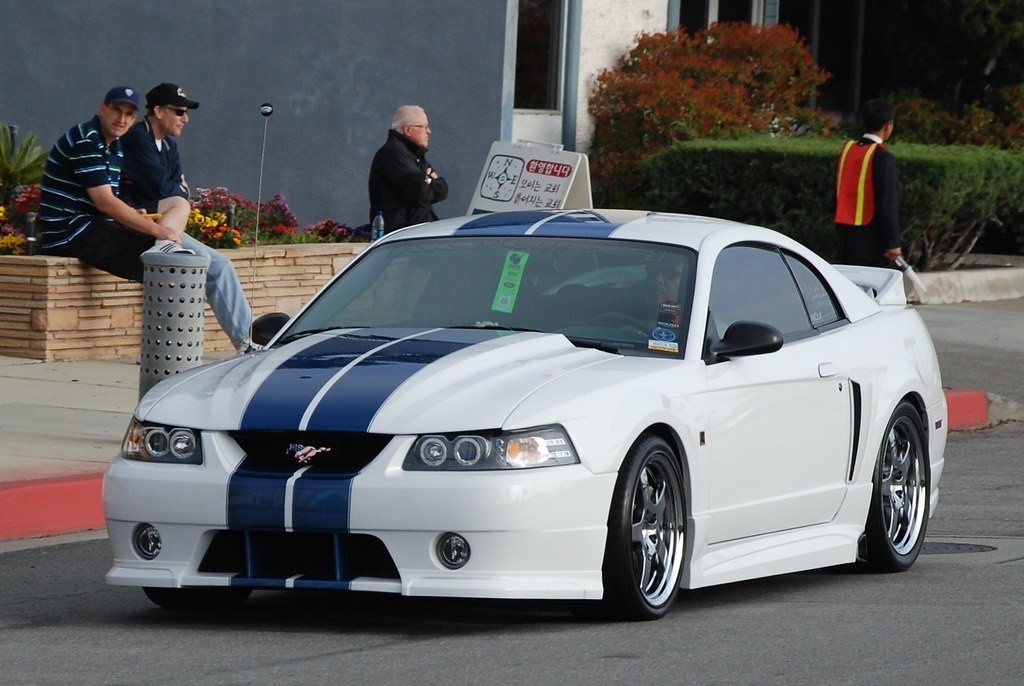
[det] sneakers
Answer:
[239,342,266,355]
[140,238,195,256]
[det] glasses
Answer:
[168,107,188,117]
[402,124,429,130]
[107,104,138,121]
[645,263,682,280]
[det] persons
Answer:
[120,81,267,358]
[366,103,450,238]
[33,80,196,289]
[609,247,687,337]
[828,98,903,270]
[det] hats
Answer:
[145,82,199,110]
[104,85,140,112]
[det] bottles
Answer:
[371,211,384,244]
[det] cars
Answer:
[103,207,946,623]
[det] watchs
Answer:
[426,177,432,185]
